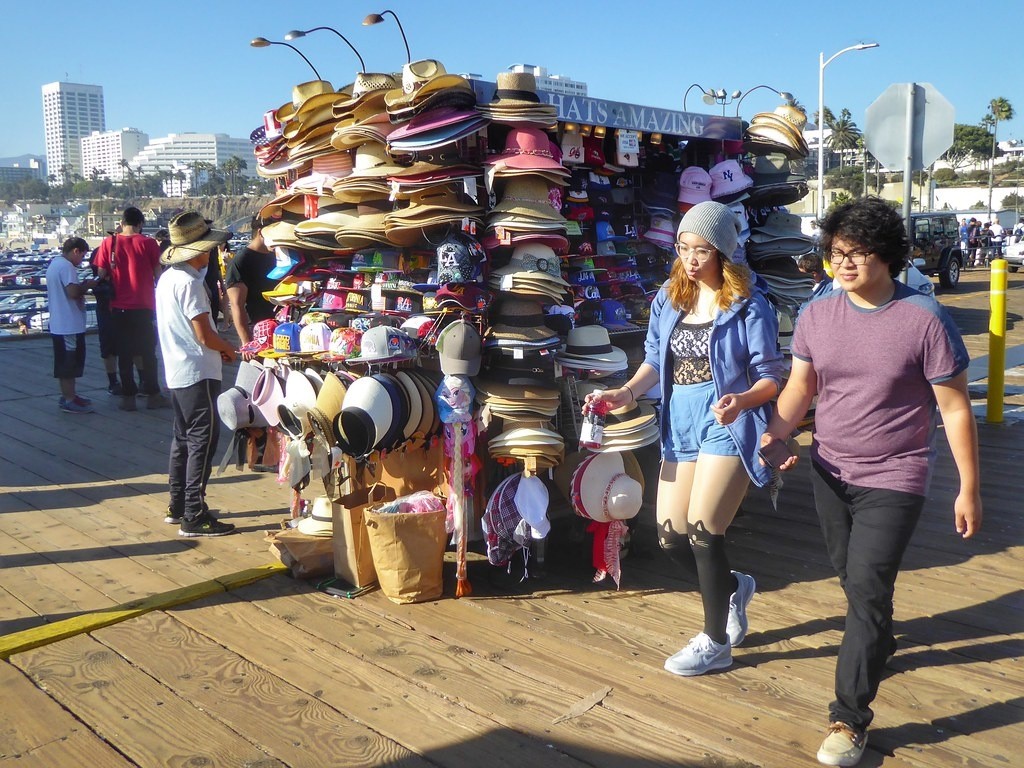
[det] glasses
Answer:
[826,248,877,265]
[675,244,716,263]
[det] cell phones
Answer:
[757,437,795,468]
[94,276,100,281]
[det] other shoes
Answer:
[116,395,138,411]
[61,401,94,414]
[223,313,233,328]
[138,383,151,396]
[107,380,121,395]
[219,325,229,333]
[57,394,91,406]
[147,393,171,409]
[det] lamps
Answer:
[564,122,577,134]
[362,10,410,63]
[579,124,591,136]
[594,126,606,138]
[736,85,794,116]
[546,123,558,132]
[650,132,661,144]
[683,83,717,113]
[285,27,366,74]
[250,38,321,81]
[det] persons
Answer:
[760,195,982,767]
[581,201,781,676]
[787,220,834,331]
[959,217,1024,267]
[46,207,285,535]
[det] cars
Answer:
[0,246,98,337]
[1004,236,1024,273]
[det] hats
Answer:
[217,57,816,589]
[107,225,143,235]
[160,212,234,266]
[677,201,742,260]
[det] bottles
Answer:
[578,389,608,449]
[301,500,313,518]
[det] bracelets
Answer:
[623,386,633,401]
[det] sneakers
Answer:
[179,514,236,538]
[816,721,869,766]
[727,571,756,648]
[664,631,733,675]
[165,501,209,523]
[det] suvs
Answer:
[902,212,965,288]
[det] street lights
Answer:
[818,41,882,254]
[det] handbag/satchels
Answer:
[363,484,447,604]
[93,277,116,301]
[331,475,397,588]
